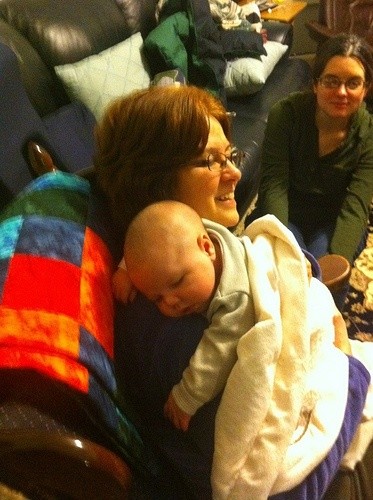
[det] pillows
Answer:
[52,33,150,129]
[217,38,290,96]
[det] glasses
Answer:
[317,76,363,90]
[205,146,242,173]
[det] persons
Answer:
[93,85,373,500]
[258,34,373,269]
[113,200,338,429]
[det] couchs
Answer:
[0,171,172,500]
[1,1,318,232]
